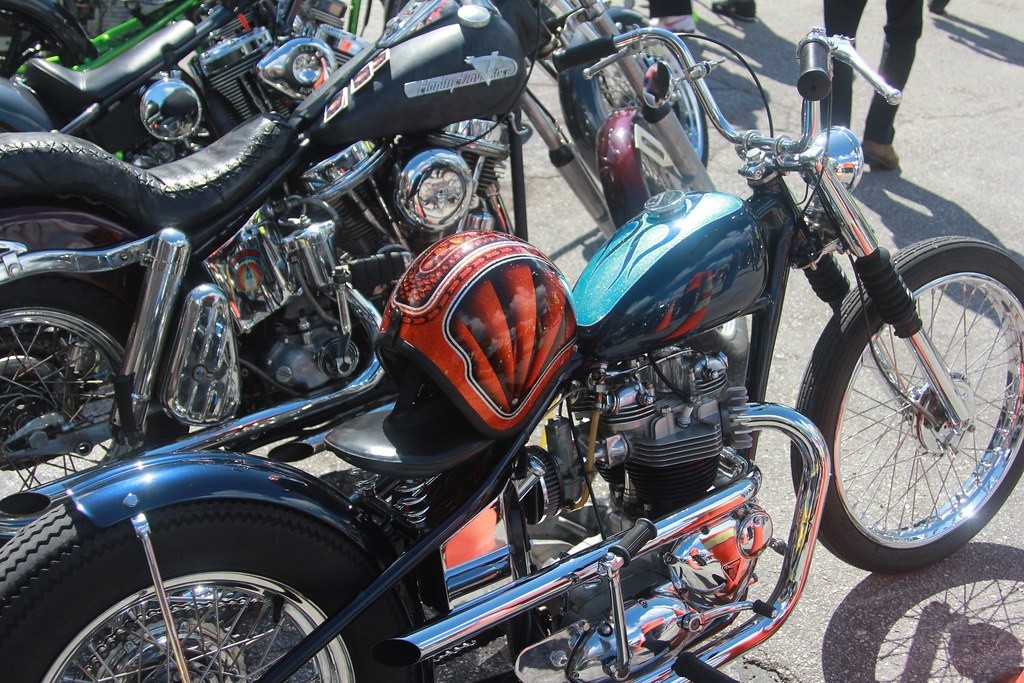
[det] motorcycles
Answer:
[1,0,752,430]
[1,26,1023,682]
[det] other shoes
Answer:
[712,0,755,22]
[861,139,898,169]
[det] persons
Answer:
[820,0,923,169]
[632,0,696,33]
[713,0,756,21]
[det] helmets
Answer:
[375,231,582,439]
